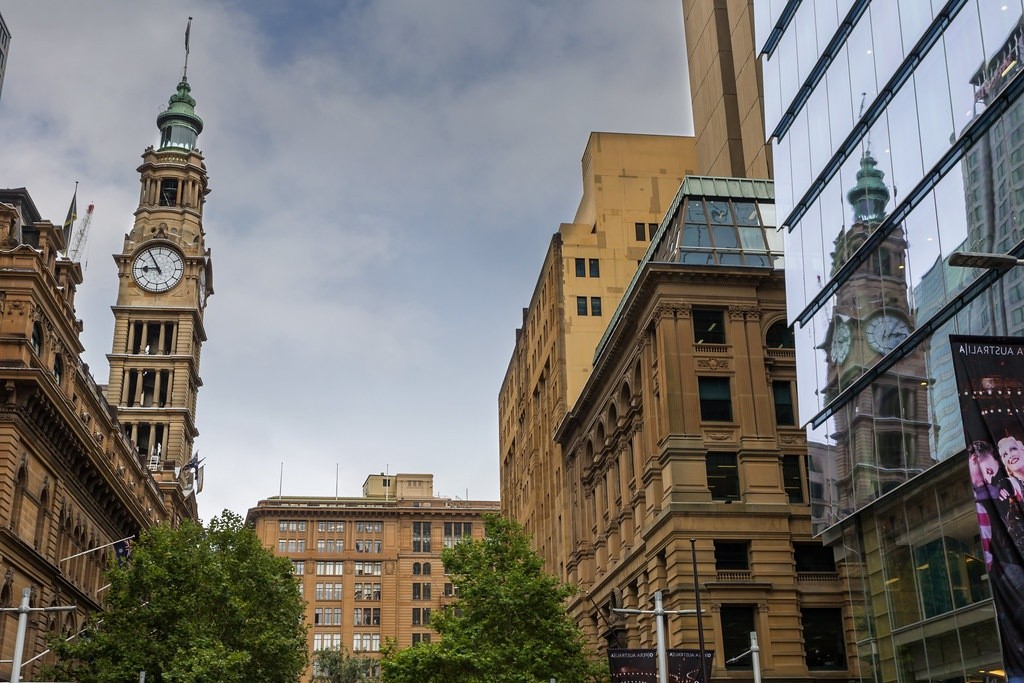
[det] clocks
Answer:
[864,315,916,358]
[131,244,186,293]
[830,323,852,364]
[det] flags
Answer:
[180,452,204,493]
[57,192,78,257]
[112,539,130,568]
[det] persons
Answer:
[968,436,1024,571]
